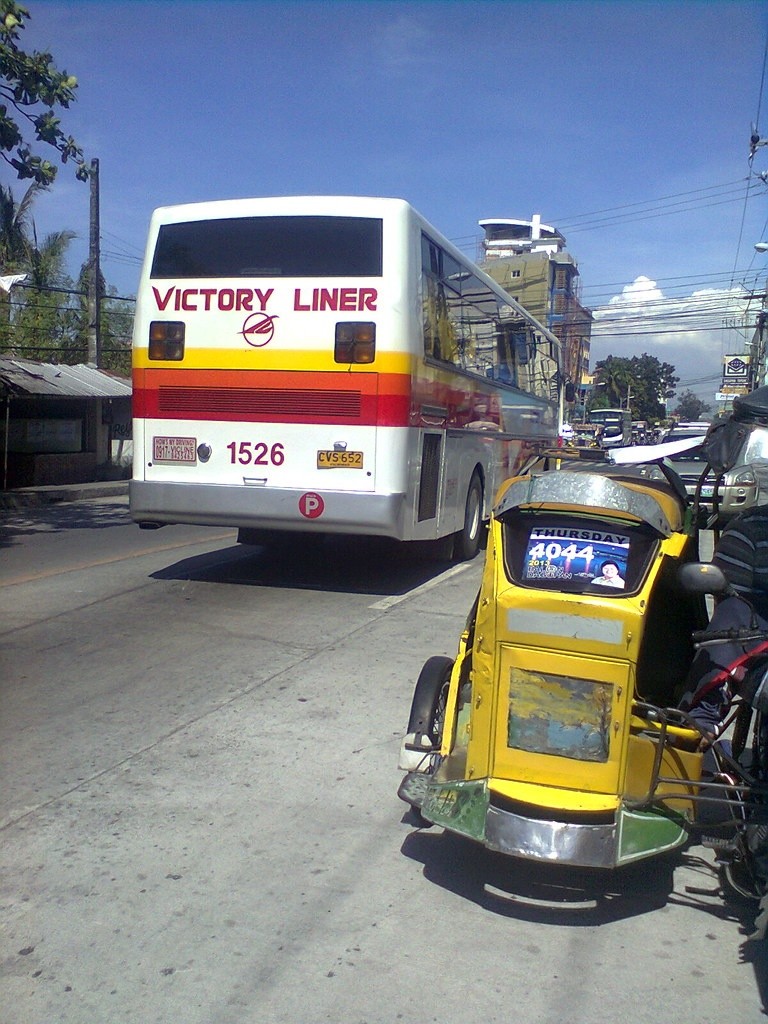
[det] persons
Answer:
[591,560,625,589]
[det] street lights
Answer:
[618,396,635,408]
[582,381,606,424]
[627,375,642,409]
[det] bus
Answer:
[587,407,632,448]
[126,194,577,562]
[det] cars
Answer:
[535,419,768,528]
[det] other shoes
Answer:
[726,826,765,900]
